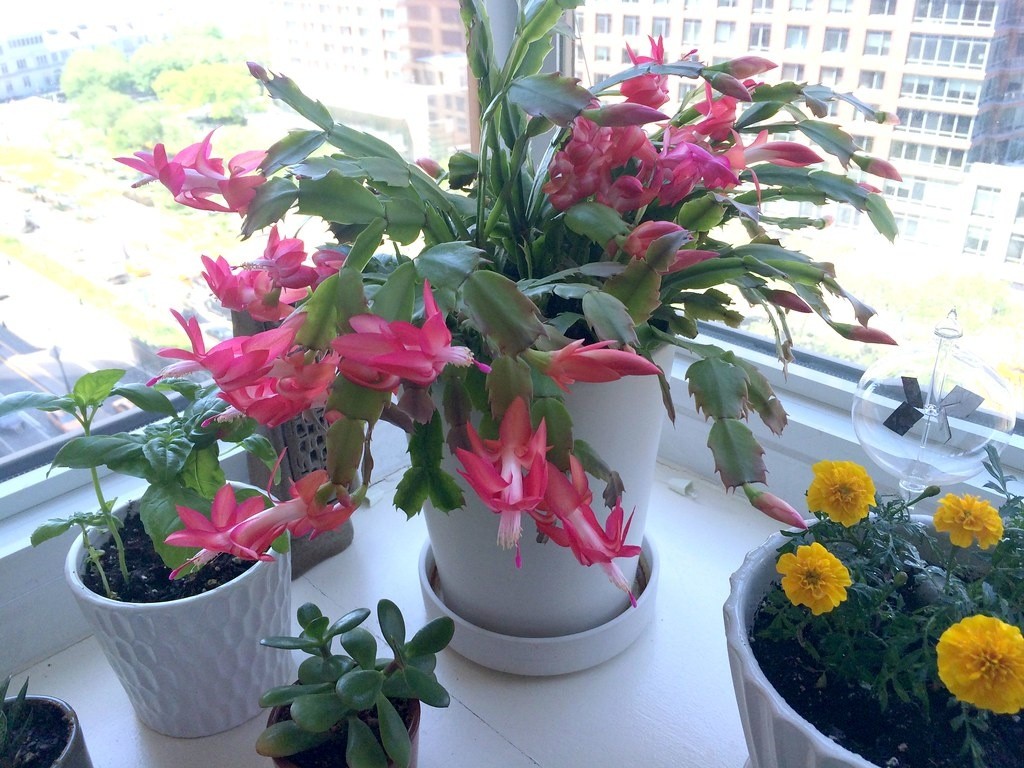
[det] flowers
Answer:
[0,341,290,598]
[755,457,1024,768]
[110,0,902,608]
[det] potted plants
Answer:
[256,599,456,768]
[0,675,92,768]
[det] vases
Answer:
[721,514,1024,767]
[64,479,291,740]
[418,313,673,674]
